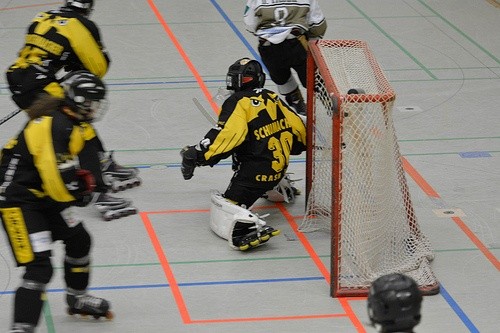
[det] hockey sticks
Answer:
[312,142,346,149]
[0,106,24,126]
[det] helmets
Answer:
[65,0,95,14]
[60,71,105,123]
[226,58,266,91]
[367,273,421,325]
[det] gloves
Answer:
[181,145,200,180]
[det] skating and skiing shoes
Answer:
[66,293,113,321]
[327,105,349,118]
[261,187,301,200]
[292,99,306,116]
[98,150,141,193]
[89,191,137,221]
[238,224,280,251]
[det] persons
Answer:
[244,0,333,116]
[6,0,142,220]
[368,274,422,333]
[180,58,307,251]
[0,73,115,333]
